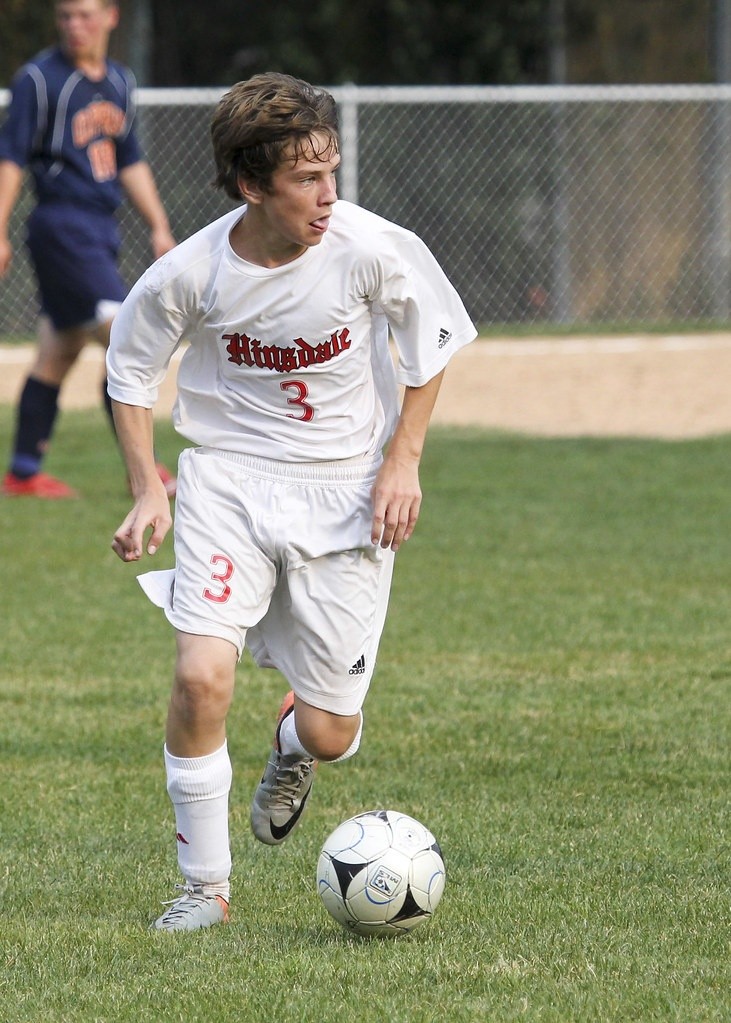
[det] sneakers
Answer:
[156,460,178,498]
[249,688,320,845]
[1,470,75,500]
[150,889,230,932]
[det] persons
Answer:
[96,71,485,934]
[1,1,181,497]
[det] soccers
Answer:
[315,809,446,940]
